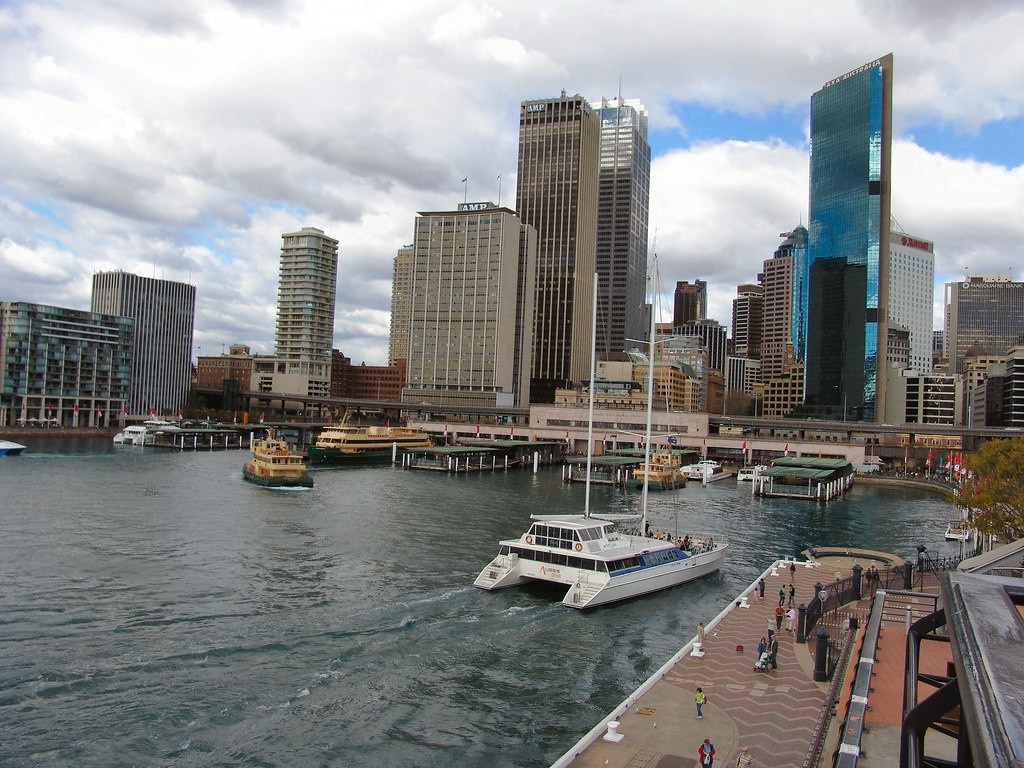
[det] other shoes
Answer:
[697,716,702,720]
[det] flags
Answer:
[743,441,746,454]
[784,445,787,456]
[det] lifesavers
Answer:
[526,536,532,543]
[575,543,582,551]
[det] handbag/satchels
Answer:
[701,693,707,704]
[779,589,784,595]
[704,754,711,765]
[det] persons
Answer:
[808,545,817,558]
[753,577,765,600]
[764,614,774,641]
[869,466,953,481]
[736,747,753,768]
[643,520,713,556]
[790,562,796,576]
[697,622,705,650]
[694,687,705,719]
[776,584,796,632]
[755,635,778,670]
[698,739,715,768]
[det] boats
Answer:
[112,425,154,446]
[0,440,27,455]
[242,429,315,489]
[736,466,769,482]
[306,425,434,466]
[470,515,731,608]
[556,445,727,493]
